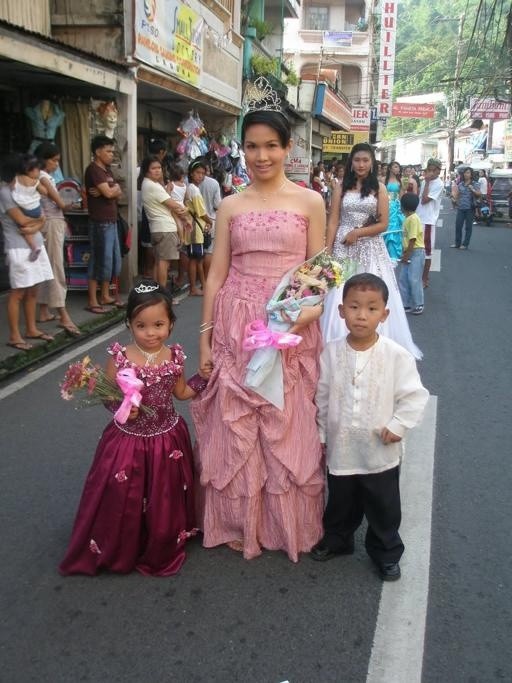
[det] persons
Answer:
[326,143,424,361]
[85,136,122,313]
[184,156,222,288]
[382,162,405,259]
[182,161,213,296]
[398,193,426,315]
[416,159,444,288]
[137,155,193,288]
[449,167,480,250]
[34,143,79,337]
[400,166,425,197]
[0,148,54,352]
[312,160,345,208]
[382,164,388,184]
[472,169,488,217]
[189,74,326,564]
[10,155,47,263]
[309,273,429,581]
[58,278,213,576]
[374,160,382,182]
[25,99,66,181]
[137,139,168,280]
[167,164,190,250]
[445,165,460,197]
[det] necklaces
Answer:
[253,182,287,201]
[345,334,376,386]
[134,342,164,363]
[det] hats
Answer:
[427,158,442,169]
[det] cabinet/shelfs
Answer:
[64,209,117,291]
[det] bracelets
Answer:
[106,401,123,414]
[200,320,214,328]
[187,373,208,393]
[200,326,214,334]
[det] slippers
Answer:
[7,298,125,349]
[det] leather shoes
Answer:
[312,541,334,559]
[377,563,401,580]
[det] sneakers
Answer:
[449,244,458,247]
[459,246,467,250]
[414,305,424,314]
[404,307,412,312]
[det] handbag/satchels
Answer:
[117,212,131,257]
[203,230,211,248]
